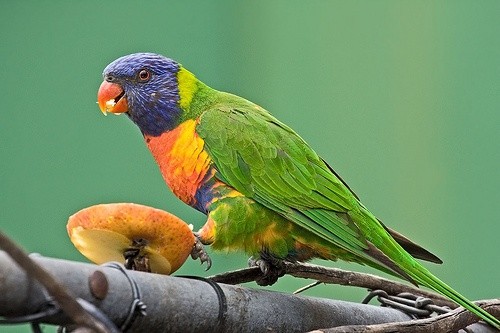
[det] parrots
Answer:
[98,52,500,332]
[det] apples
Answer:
[64,202,195,276]
